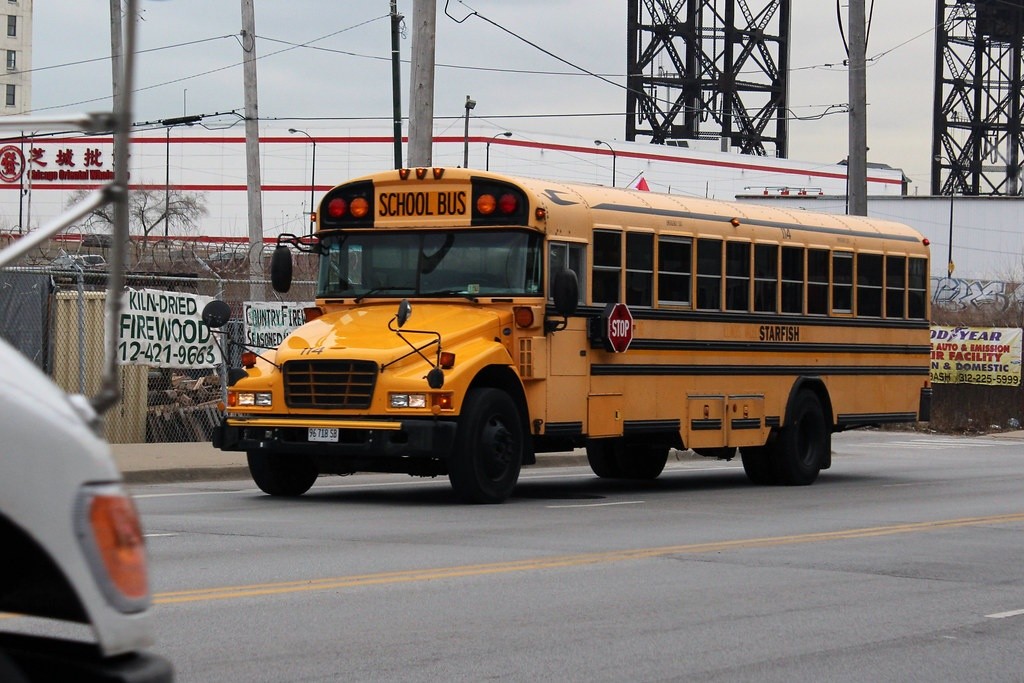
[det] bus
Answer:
[209,165,934,503]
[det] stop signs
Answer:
[607,302,635,355]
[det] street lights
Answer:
[934,155,957,280]
[462,92,477,168]
[594,139,616,189]
[485,130,513,171]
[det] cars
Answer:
[0,329,175,683]
